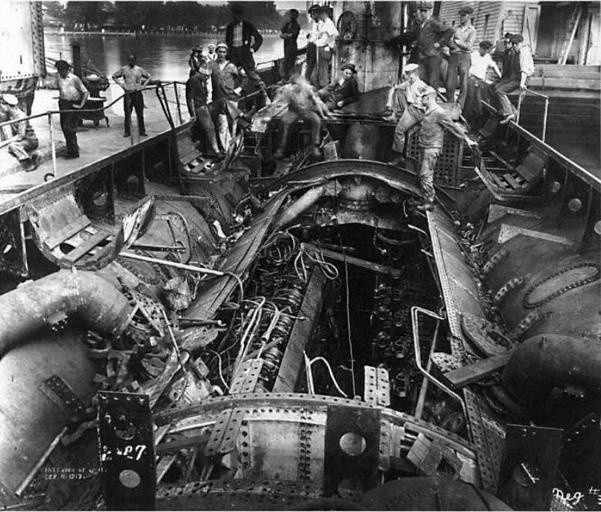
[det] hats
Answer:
[402,63,420,71]
[341,63,357,74]
[230,4,245,12]
[458,6,473,14]
[510,34,524,43]
[417,3,432,11]
[215,43,229,50]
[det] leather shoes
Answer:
[140,132,148,137]
[122,132,131,137]
[25,163,39,172]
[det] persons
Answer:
[54,60,90,159]
[1,92,43,173]
[382,5,537,213]
[111,53,155,139]
[187,5,360,161]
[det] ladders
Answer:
[557,2,583,66]
